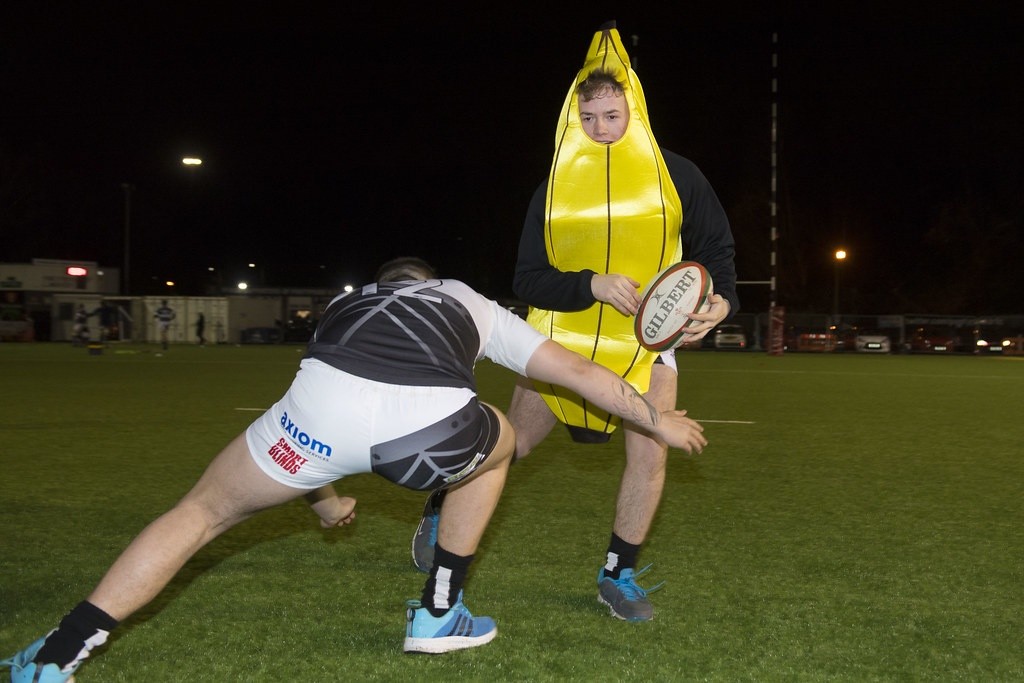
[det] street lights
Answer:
[834,250,846,314]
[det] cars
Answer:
[714,322,747,351]
[857,328,891,353]
[913,328,958,354]
[977,331,1024,356]
[785,325,853,352]
[277,305,319,341]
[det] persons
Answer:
[74,304,88,342]
[0,258,707,683]
[87,301,116,341]
[152,299,175,346]
[411,26,741,623]
[193,312,205,344]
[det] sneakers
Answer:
[403,588,497,654]
[410,488,445,570]
[0,637,82,683]
[598,562,666,621]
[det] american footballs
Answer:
[634,261,714,354]
